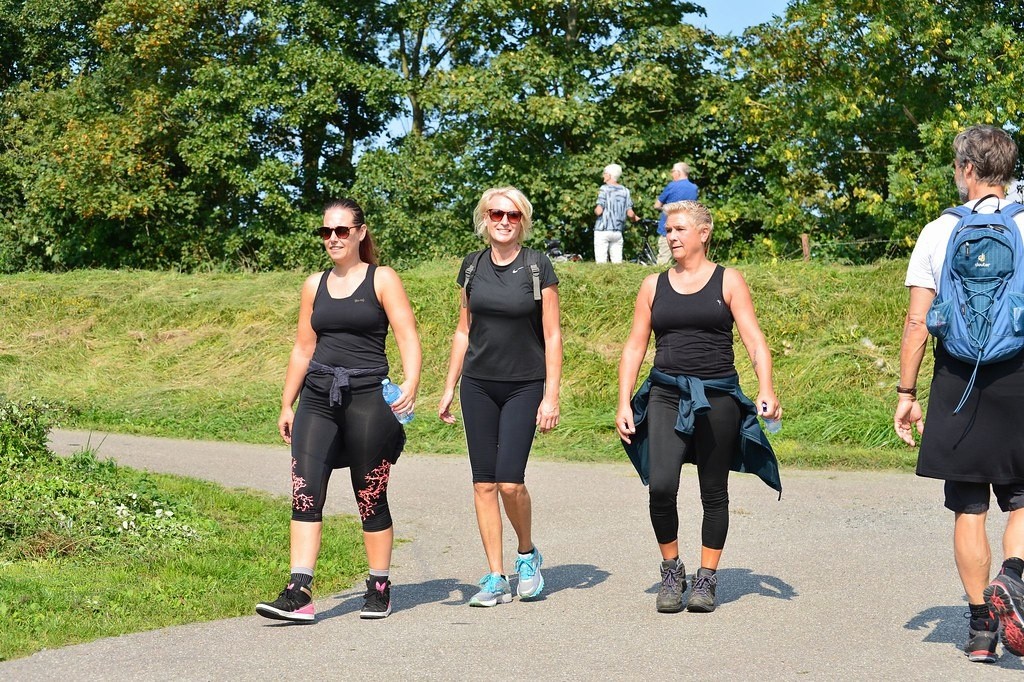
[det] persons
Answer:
[255,199,422,623]
[614,200,782,613]
[893,125,1024,663]
[594,164,641,263]
[654,162,698,265]
[437,186,563,607]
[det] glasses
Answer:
[318,225,360,240]
[486,208,522,224]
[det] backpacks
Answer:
[926,193,1024,365]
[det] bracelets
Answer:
[896,386,916,399]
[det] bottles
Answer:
[381,379,414,424]
[760,402,782,433]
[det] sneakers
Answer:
[360,578,391,619]
[656,559,688,611]
[469,573,512,606]
[688,568,718,611]
[983,568,1024,657]
[255,574,314,621]
[963,611,998,662]
[515,543,544,597]
